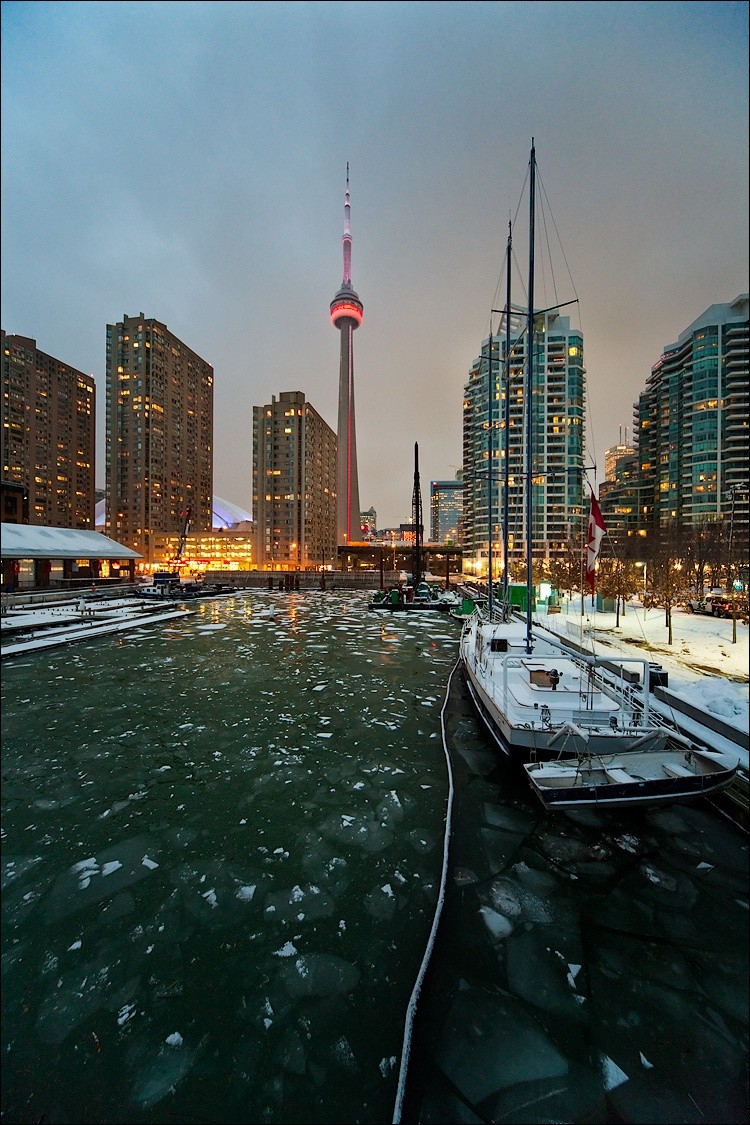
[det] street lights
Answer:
[634,561,647,594]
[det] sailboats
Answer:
[458,133,678,757]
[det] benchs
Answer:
[607,769,636,784]
[661,763,696,777]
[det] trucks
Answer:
[685,593,749,621]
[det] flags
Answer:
[585,492,608,594]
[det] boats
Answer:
[521,749,742,810]
[135,579,237,600]
